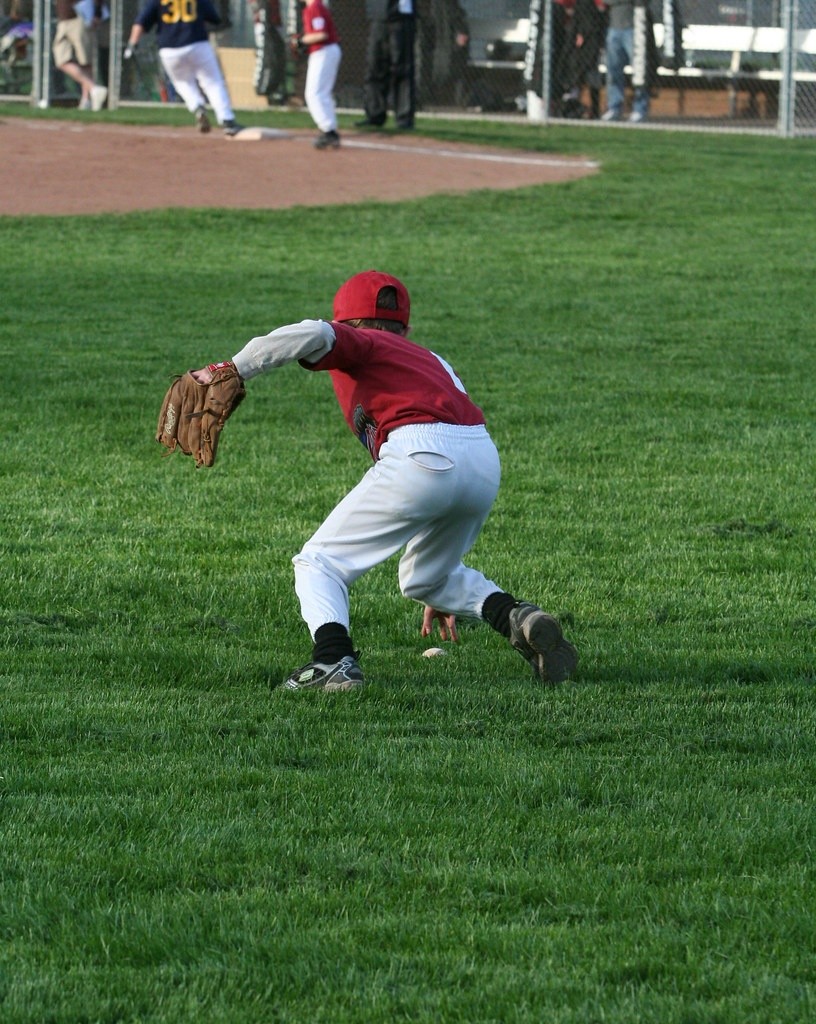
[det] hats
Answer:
[333,270,410,328]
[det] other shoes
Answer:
[601,109,620,121]
[396,119,414,130]
[78,99,90,110]
[223,121,245,136]
[354,119,384,128]
[194,105,210,134]
[314,134,341,150]
[90,85,108,112]
[629,111,647,123]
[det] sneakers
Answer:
[280,650,365,693]
[508,601,578,682]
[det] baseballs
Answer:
[422,647,446,657]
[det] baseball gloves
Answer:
[155,359,247,468]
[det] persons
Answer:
[353,0,421,134]
[122,0,245,135]
[462,39,523,113]
[522,0,605,120]
[734,61,779,126]
[250,0,291,105]
[155,270,582,692]
[600,0,653,123]
[288,0,343,149]
[0,0,110,113]
